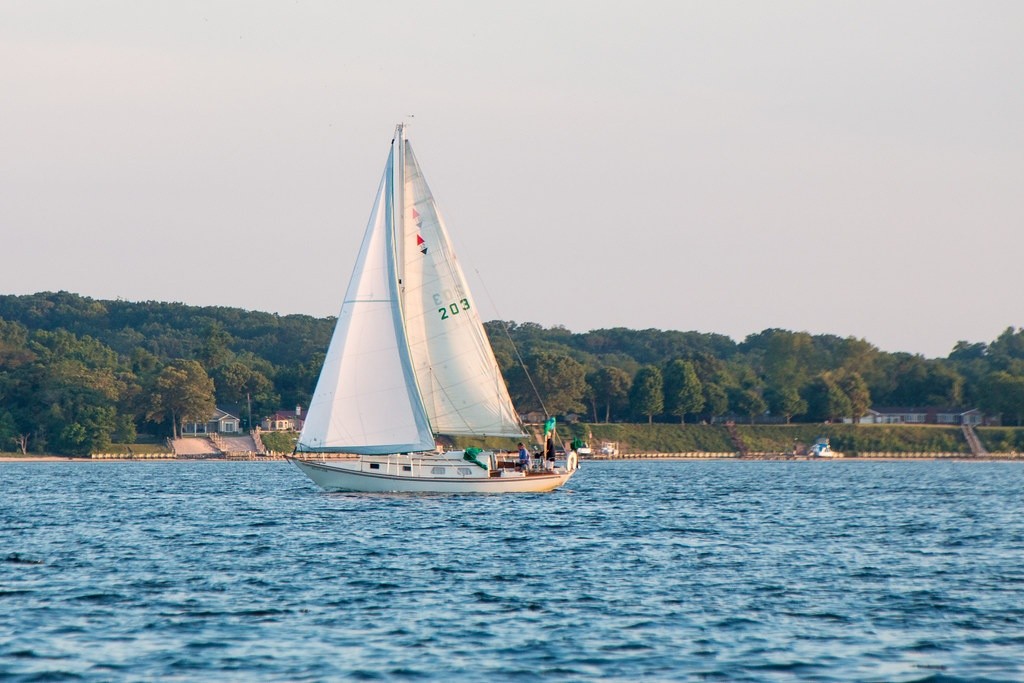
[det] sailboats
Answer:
[282,114,583,495]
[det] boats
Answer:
[809,438,845,459]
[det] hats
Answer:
[543,432,552,437]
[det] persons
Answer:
[547,431,555,462]
[518,442,532,473]
